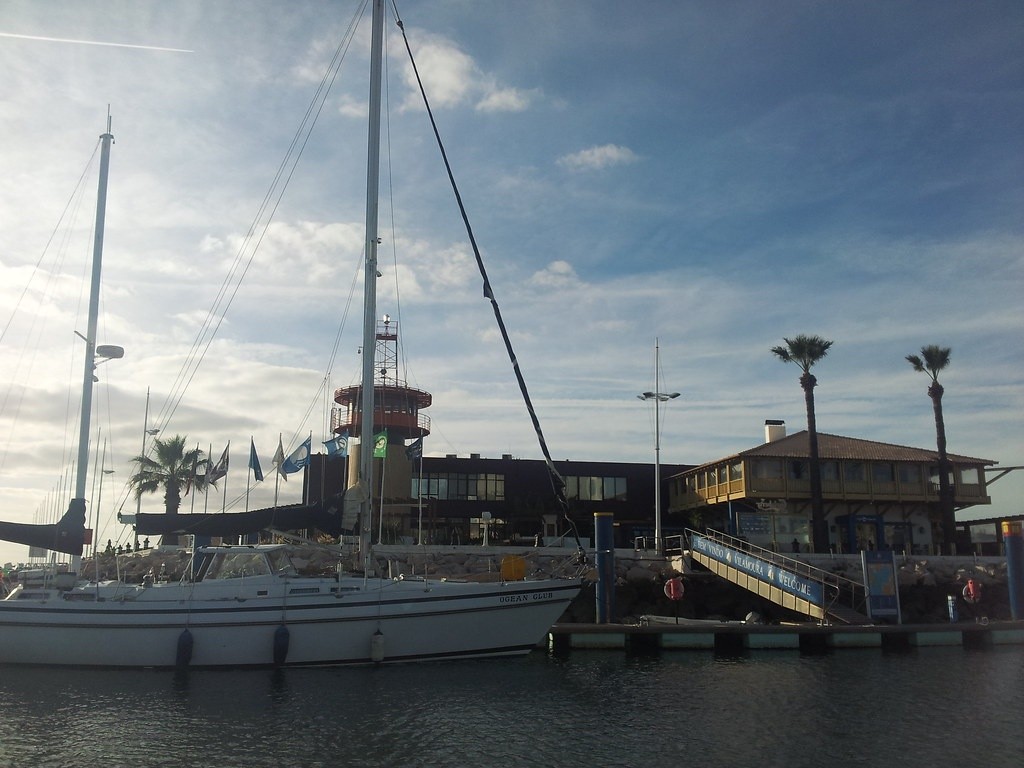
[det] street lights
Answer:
[637,336,681,556]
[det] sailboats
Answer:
[0,0,599,671]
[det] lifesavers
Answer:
[962,584,982,604]
[664,578,685,601]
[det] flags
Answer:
[373,430,387,458]
[281,436,311,473]
[272,440,287,482]
[184,459,196,496]
[249,440,263,482]
[405,436,422,460]
[322,431,348,458]
[205,455,218,492]
[210,443,229,483]
